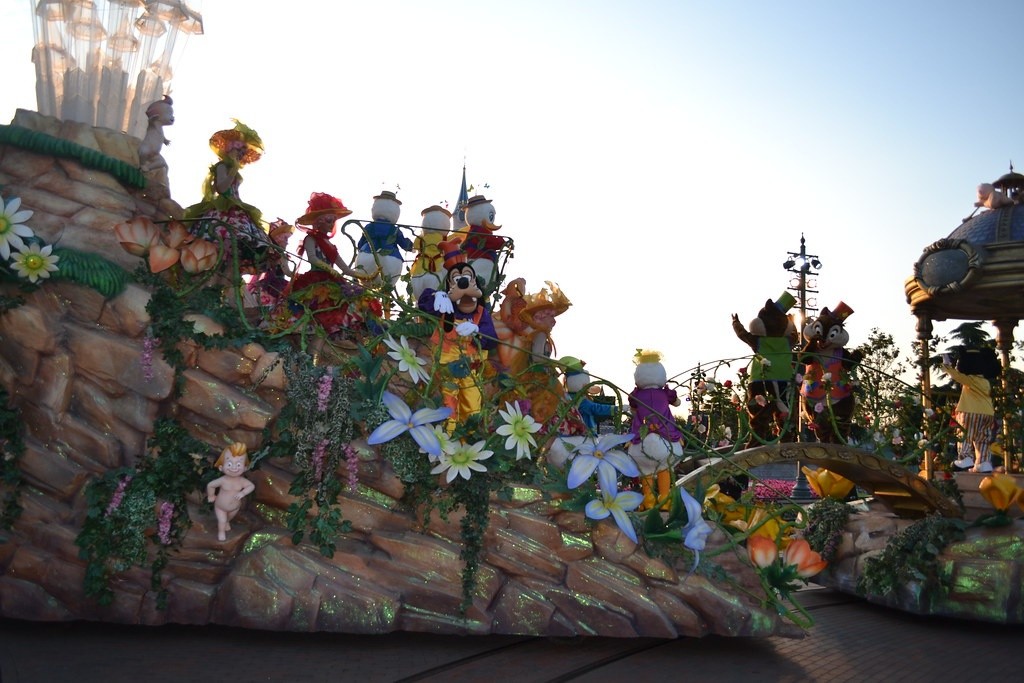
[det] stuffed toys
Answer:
[557,356,630,438]
[797,302,863,445]
[355,191,414,319]
[940,348,993,472]
[410,205,451,299]
[732,292,797,448]
[461,195,506,292]
[627,349,684,509]
[418,238,499,445]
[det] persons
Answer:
[258,193,382,332]
[206,442,255,540]
[517,287,587,434]
[249,219,295,305]
[185,123,280,272]
[140,95,175,172]
